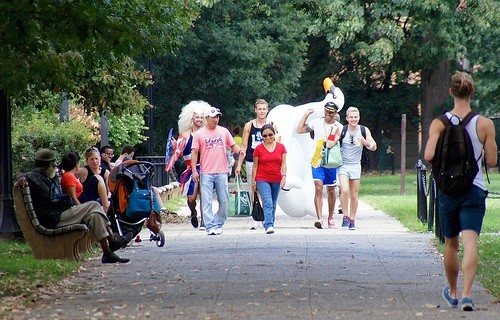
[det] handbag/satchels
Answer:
[126,175,161,217]
[251,191,264,221]
[322,122,343,169]
[234,173,251,216]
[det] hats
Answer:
[204,107,222,118]
[324,101,338,113]
[35,148,56,161]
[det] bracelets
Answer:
[282,175,286,177]
[366,142,373,150]
[239,150,242,154]
[252,184,256,187]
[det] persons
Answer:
[16,148,134,264]
[59,145,167,243]
[252,124,287,234]
[334,106,378,230]
[170,99,212,230]
[235,99,282,230]
[191,107,246,235]
[296,102,346,229]
[424,71,498,311]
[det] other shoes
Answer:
[267,227,274,234]
[216,227,222,235]
[314,220,323,229]
[135,239,141,242]
[338,205,343,214]
[109,232,133,252]
[208,229,216,235]
[102,254,130,264]
[250,221,260,229]
[191,210,199,228]
[328,218,335,228]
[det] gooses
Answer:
[263,77,346,217]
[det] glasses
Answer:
[86,146,98,152]
[349,134,354,144]
[105,152,115,158]
[208,115,219,118]
[261,133,274,138]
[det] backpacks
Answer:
[432,110,485,197]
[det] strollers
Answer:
[108,161,166,248]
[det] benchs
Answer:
[12,180,95,262]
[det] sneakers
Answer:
[349,219,355,229]
[342,215,349,227]
[462,298,475,311]
[442,284,458,308]
